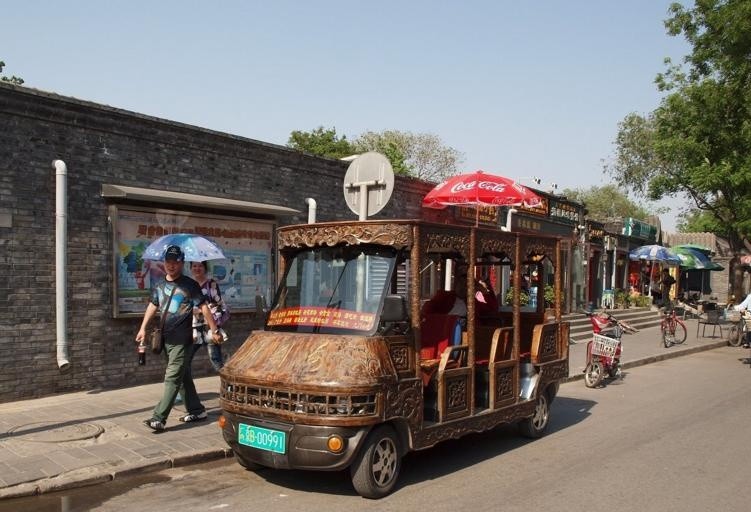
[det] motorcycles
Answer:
[580,306,639,387]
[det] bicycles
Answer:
[660,297,688,348]
[726,310,748,346]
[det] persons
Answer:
[659,268,677,313]
[422,267,500,329]
[737,293,751,348]
[174,260,227,402]
[136,244,226,431]
[691,292,720,311]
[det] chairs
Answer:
[372,295,411,335]
[697,310,723,340]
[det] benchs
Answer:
[420,312,547,423]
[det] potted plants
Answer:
[616,292,651,309]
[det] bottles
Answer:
[136,339,148,367]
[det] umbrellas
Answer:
[672,245,713,270]
[422,170,544,228]
[688,261,726,300]
[140,233,227,264]
[629,244,684,296]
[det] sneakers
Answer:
[179,411,208,423]
[142,418,164,432]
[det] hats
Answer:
[164,245,184,261]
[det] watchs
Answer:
[212,329,220,335]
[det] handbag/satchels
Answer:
[150,327,163,354]
[206,279,231,326]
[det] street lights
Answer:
[601,254,607,291]
